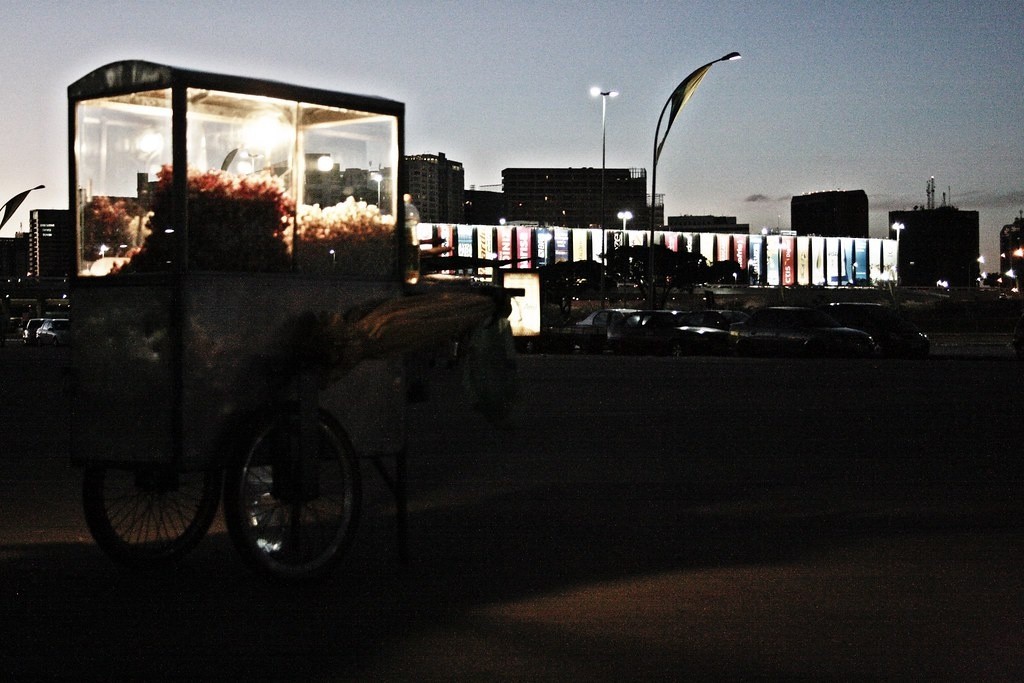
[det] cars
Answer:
[520,300,932,362]
[21,316,71,347]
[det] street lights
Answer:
[589,88,619,307]
[617,211,634,230]
[891,224,904,242]
[647,51,740,314]
